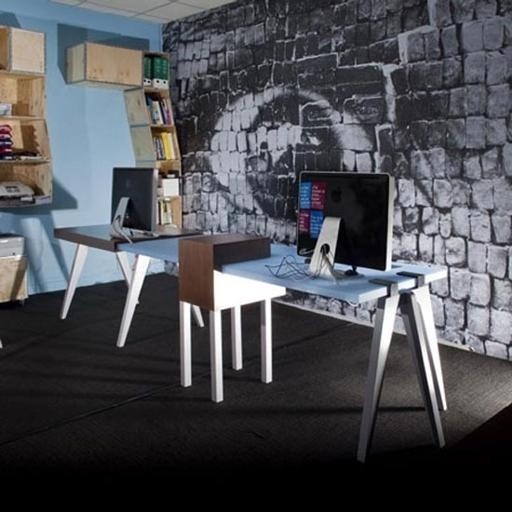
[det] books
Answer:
[145,54,182,226]
[14,150,42,160]
[0,102,14,160]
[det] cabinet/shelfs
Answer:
[0,26,53,209]
[66,41,142,91]
[123,51,183,229]
[0,255,28,306]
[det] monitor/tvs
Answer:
[110,166,159,239]
[296,170,395,282]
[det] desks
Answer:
[116,235,448,462]
[53,224,204,348]
